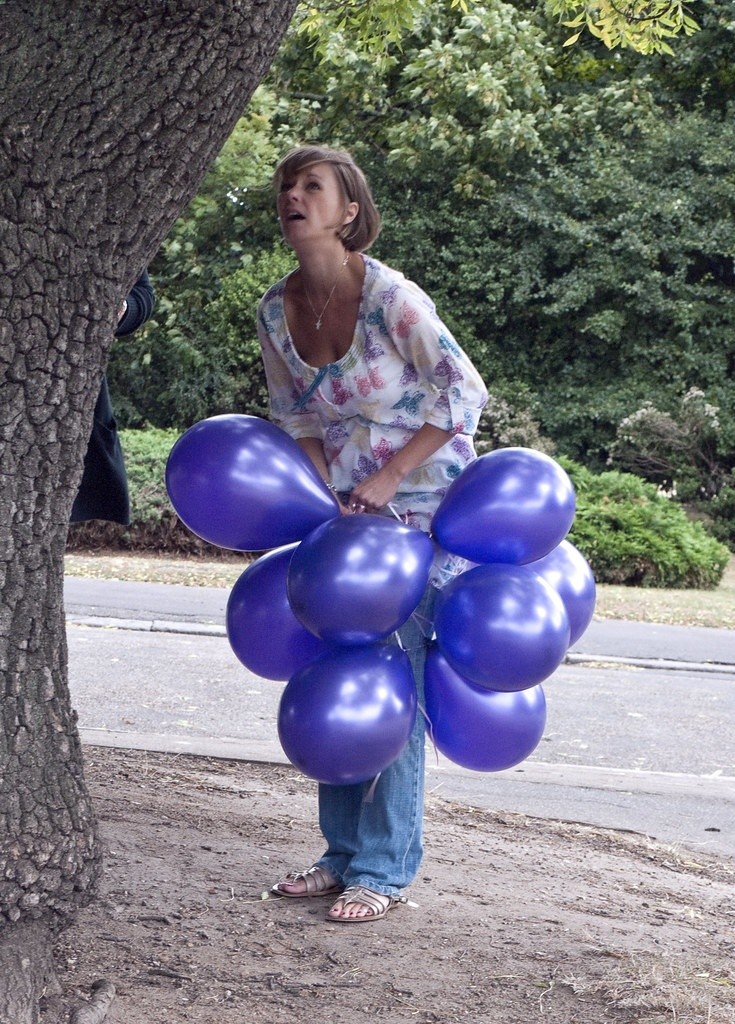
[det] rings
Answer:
[348,499,357,507]
[358,503,366,509]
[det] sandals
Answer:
[272,865,341,897]
[326,885,402,921]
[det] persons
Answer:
[258,147,490,922]
[70,265,156,523]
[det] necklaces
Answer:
[303,251,351,329]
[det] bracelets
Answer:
[324,479,338,492]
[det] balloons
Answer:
[167,415,595,784]
